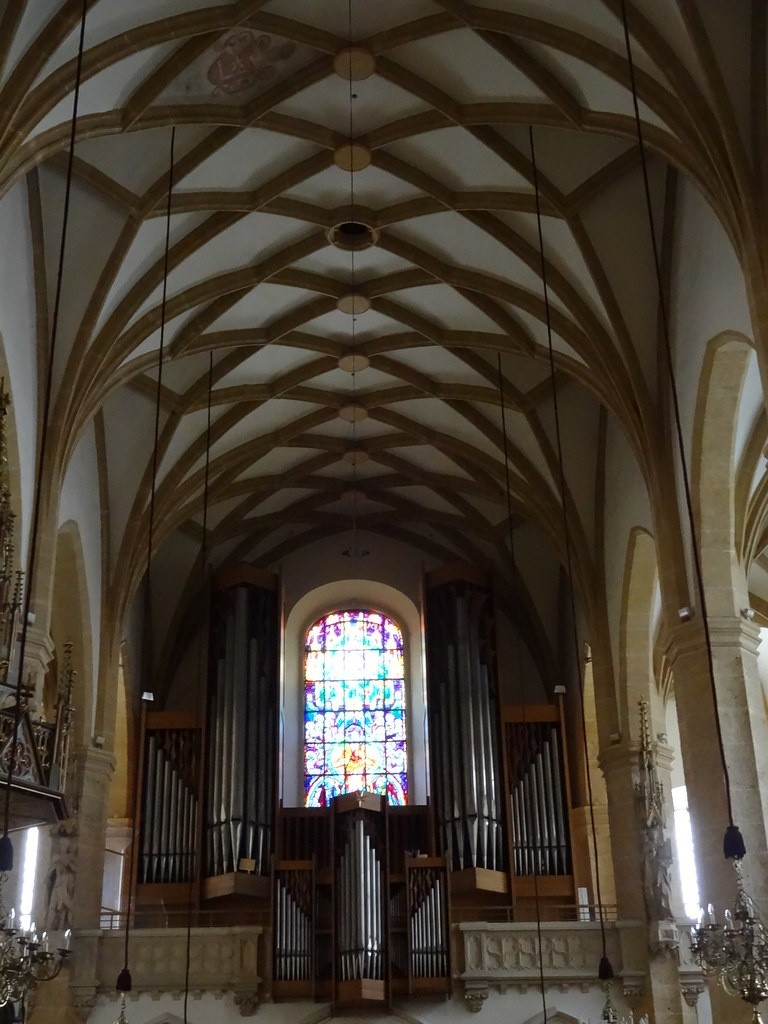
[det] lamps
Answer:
[531,1,768,1024]
[0,0,101,1024]
